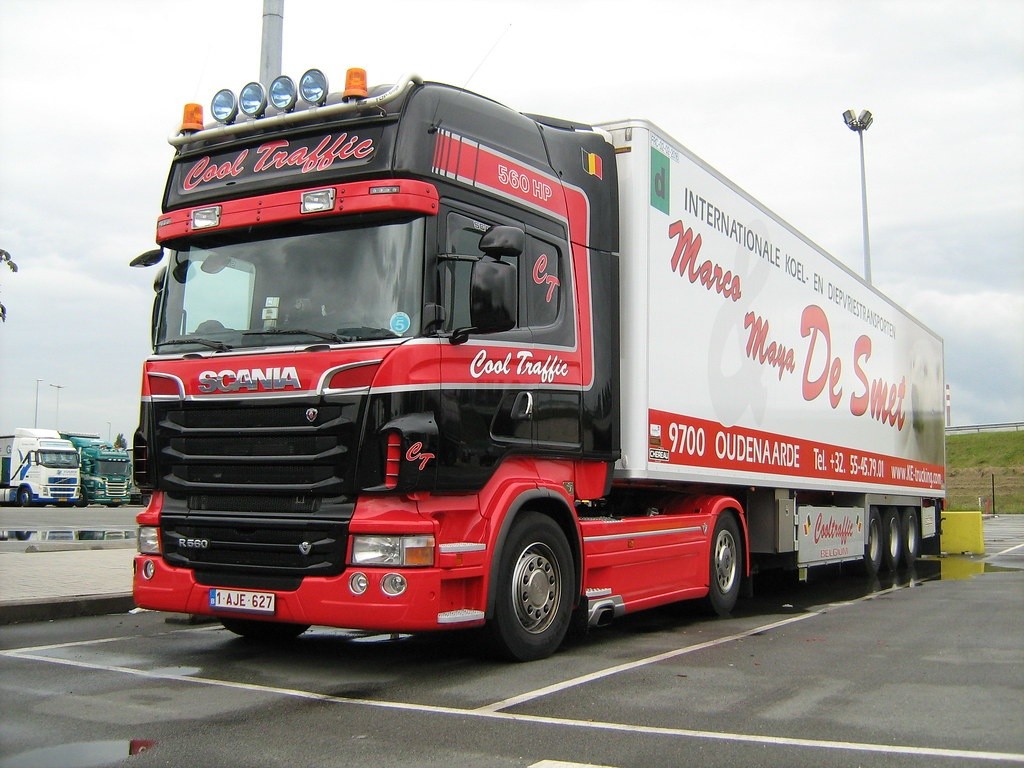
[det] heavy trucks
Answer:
[136,67,948,661]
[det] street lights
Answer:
[50,383,66,427]
[33,379,43,426]
[841,106,876,284]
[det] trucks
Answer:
[58,431,132,507]
[79,529,138,540]
[0,530,78,545]
[0,429,81,509]
